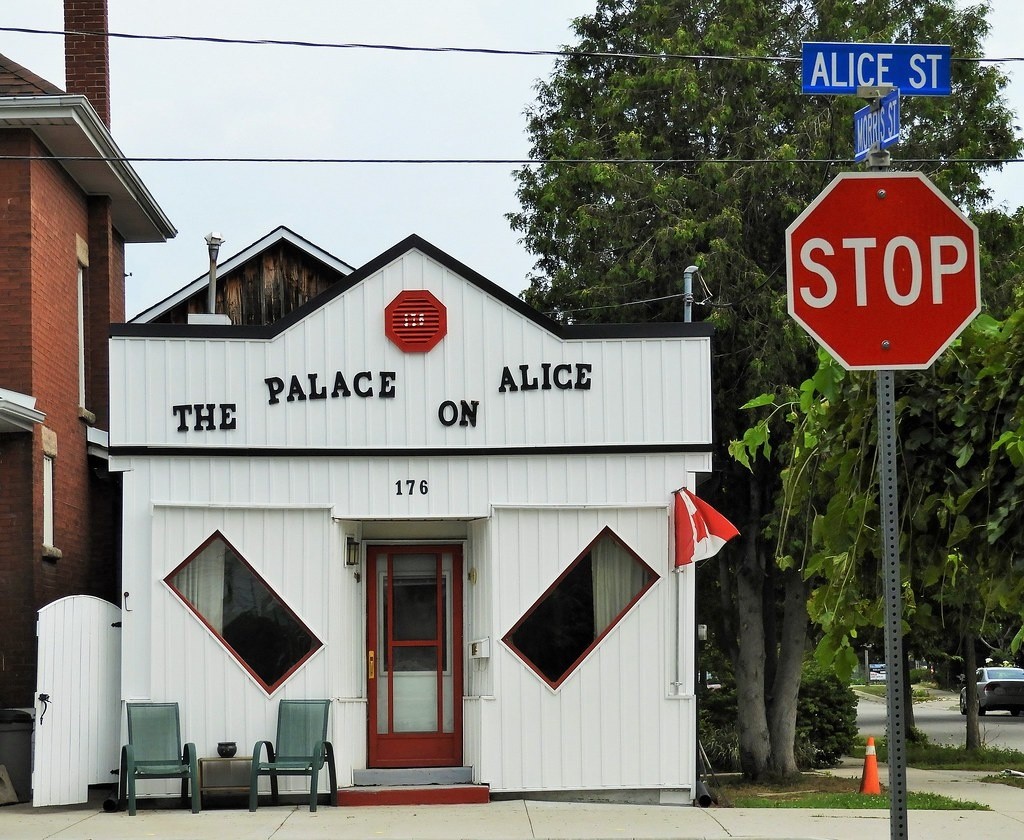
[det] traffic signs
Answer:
[802,40,952,97]
[854,89,901,161]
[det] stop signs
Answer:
[783,170,979,371]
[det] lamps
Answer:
[346,536,359,567]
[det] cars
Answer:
[959,667,1024,716]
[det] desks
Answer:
[198,757,255,809]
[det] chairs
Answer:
[118,701,200,815]
[249,698,337,812]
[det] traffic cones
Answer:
[860,737,881,795]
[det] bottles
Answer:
[217,742,237,758]
